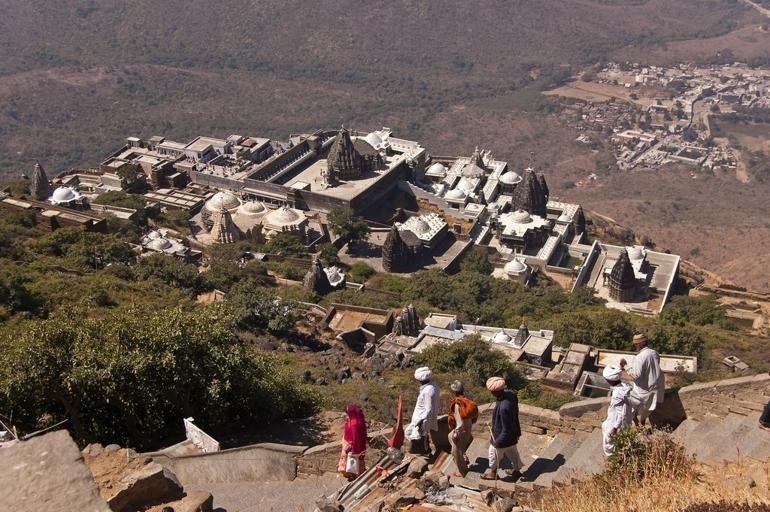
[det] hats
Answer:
[632,333,649,344]
[486,376,505,392]
[451,380,462,392]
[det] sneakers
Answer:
[480,473,496,479]
[505,468,517,475]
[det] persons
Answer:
[482,376,526,478]
[338,403,368,487]
[600,364,635,464]
[405,365,442,456]
[446,380,479,479]
[618,333,666,428]
[758,400,770,432]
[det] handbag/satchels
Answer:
[346,452,360,475]
[452,428,474,452]
